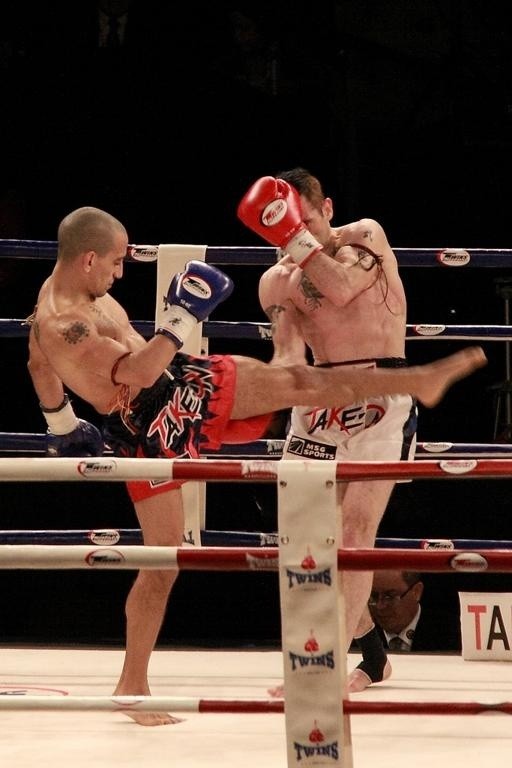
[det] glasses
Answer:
[368,582,417,607]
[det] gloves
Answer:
[237,175,324,271]
[223,412,273,445]
[157,259,235,350]
[39,394,104,458]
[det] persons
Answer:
[231,167,416,695]
[24,205,490,730]
[366,569,459,652]
